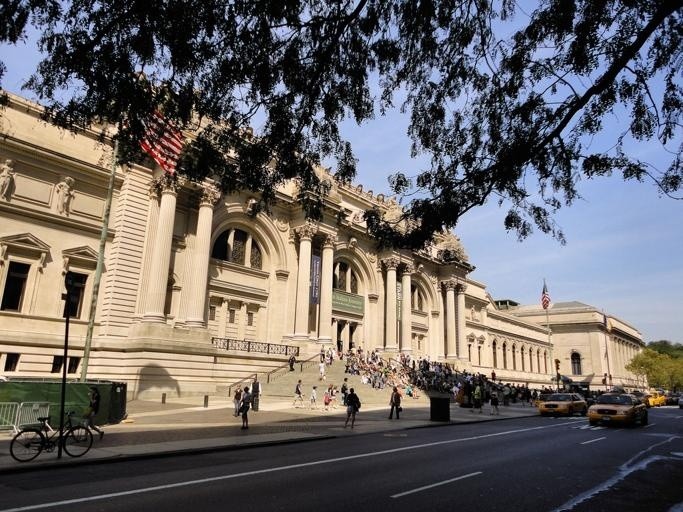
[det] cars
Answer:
[532,388,683,427]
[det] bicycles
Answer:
[11,410,92,463]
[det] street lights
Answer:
[57,270,87,461]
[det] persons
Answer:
[0,157,18,200]
[54,175,75,215]
[249,379,261,412]
[343,388,359,429]
[287,353,295,371]
[388,387,401,419]
[310,344,606,414]
[291,379,303,408]
[81,386,104,441]
[232,384,242,417]
[239,386,251,429]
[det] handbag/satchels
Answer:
[80,416,90,428]
[356,402,360,408]
[398,407,402,411]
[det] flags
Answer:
[123,104,187,178]
[540,282,550,309]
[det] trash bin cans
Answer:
[429,396,451,422]
